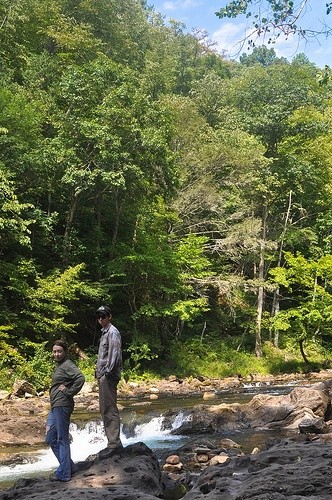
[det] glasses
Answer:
[97,315,109,319]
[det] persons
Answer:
[95,306,123,459]
[46,340,85,482]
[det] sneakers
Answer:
[49,474,59,482]
[99,445,124,459]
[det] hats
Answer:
[96,306,111,316]
